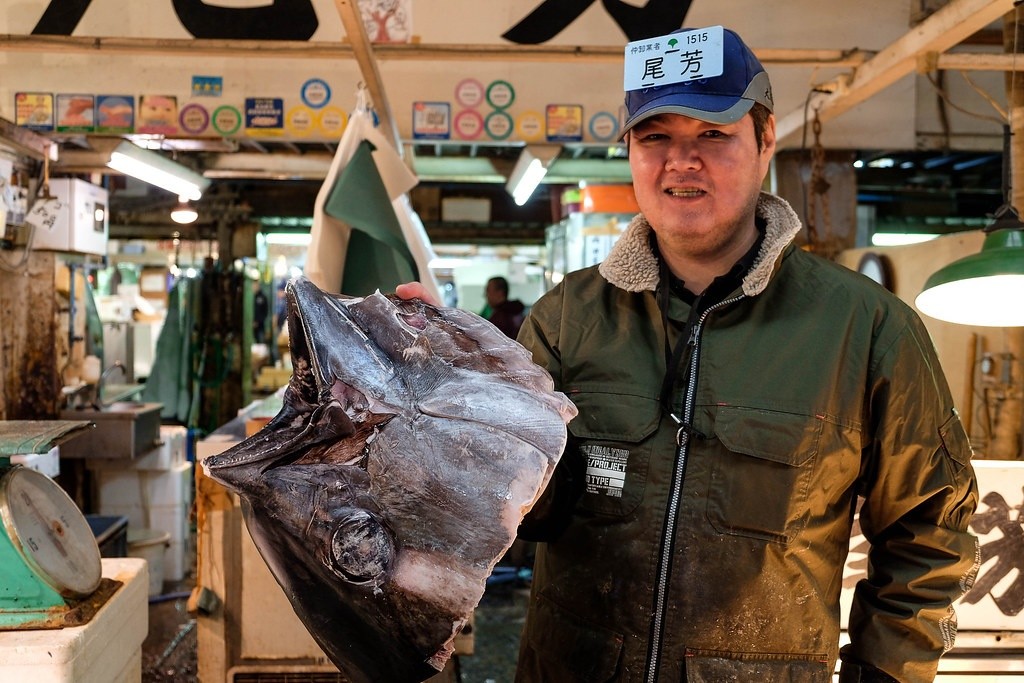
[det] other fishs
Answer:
[199,276,581,683]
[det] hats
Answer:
[617,25,773,142]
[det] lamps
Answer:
[541,159,632,184]
[914,205,1024,328]
[504,144,563,207]
[413,155,506,183]
[102,140,210,201]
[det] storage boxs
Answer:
[84,425,188,470]
[98,459,192,507]
[0,557,150,683]
[100,505,190,544]
[163,540,191,581]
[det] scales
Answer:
[0,420,124,632]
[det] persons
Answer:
[475,276,528,341]
[395,26,980,683]
